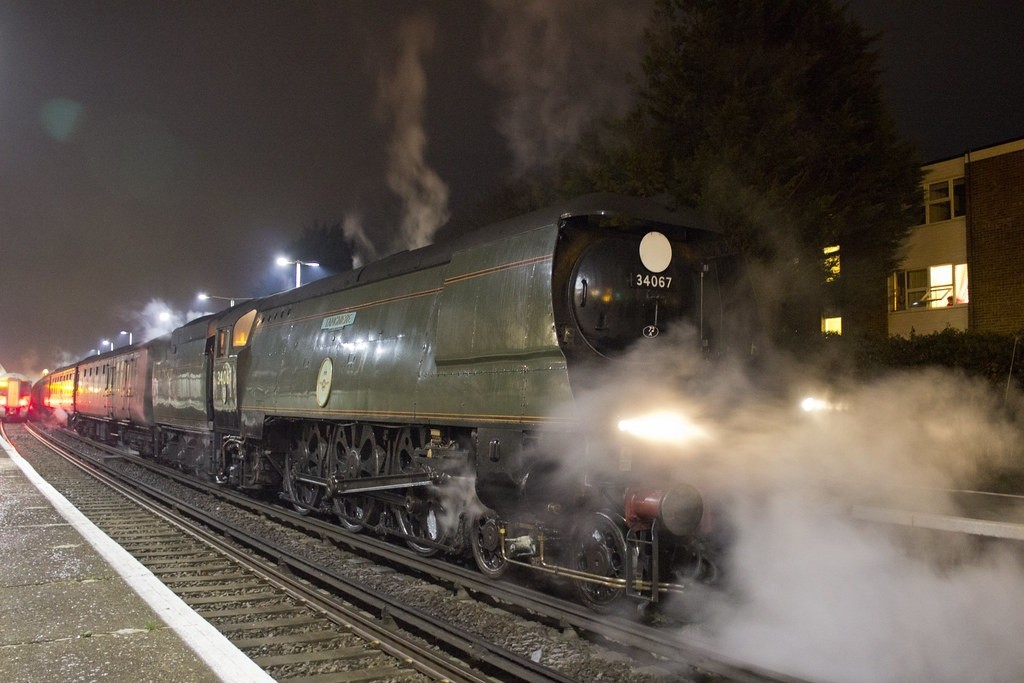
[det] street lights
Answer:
[120,330,132,346]
[276,257,320,288]
[90,349,101,354]
[102,341,113,351]
[197,293,252,308]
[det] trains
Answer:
[0,372,32,422]
[31,194,750,621]
